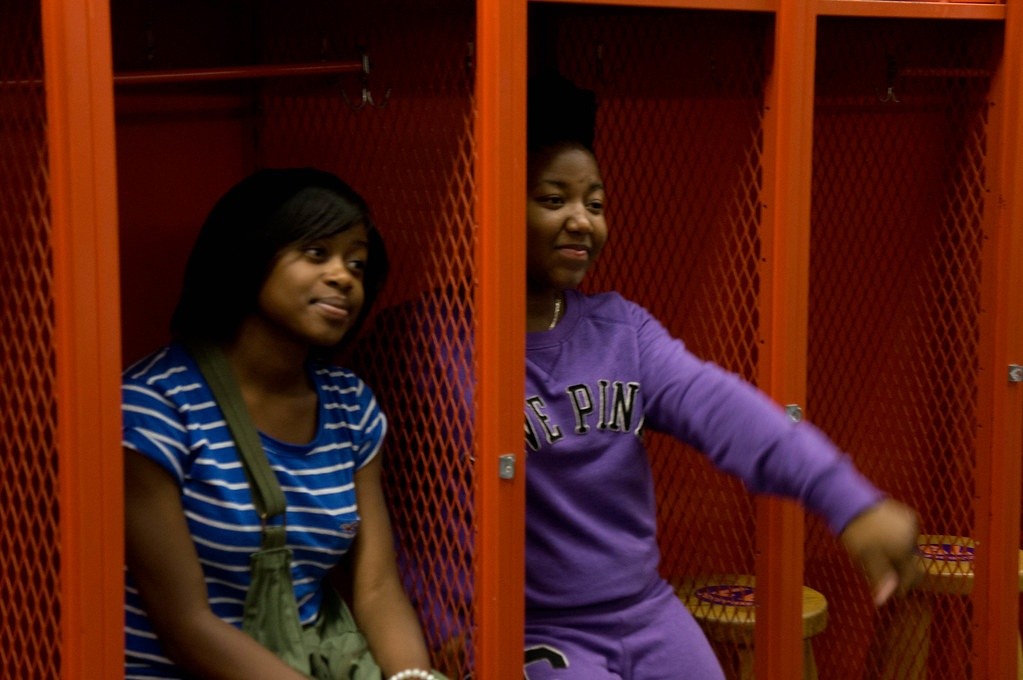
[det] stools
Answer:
[672,573,829,680]
[884,533,1023,679]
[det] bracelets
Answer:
[387,668,434,679]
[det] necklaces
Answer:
[549,301,562,334]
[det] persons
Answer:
[120,166,441,680]
[352,76,922,680]
